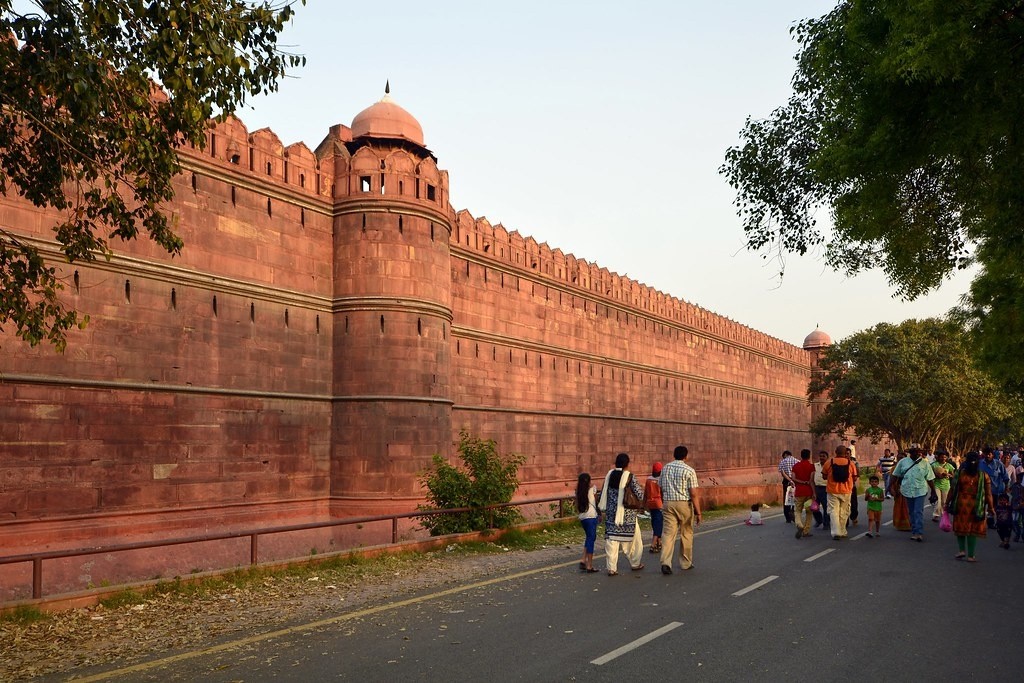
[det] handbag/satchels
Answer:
[804,497,820,513]
[897,476,904,486]
[785,485,797,506]
[622,473,646,510]
[938,504,953,532]
[596,502,602,516]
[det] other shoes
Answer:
[955,550,976,563]
[875,532,880,537]
[795,518,859,540]
[661,565,672,575]
[785,514,795,523]
[910,534,924,542]
[865,531,873,537]
[681,563,695,570]
[987,525,1024,549]
[883,494,891,499]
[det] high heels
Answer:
[579,561,602,573]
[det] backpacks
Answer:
[644,478,663,510]
[831,458,850,482]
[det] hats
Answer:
[908,443,921,450]
[652,461,662,473]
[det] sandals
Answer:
[608,570,619,577]
[632,564,644,571]
[649,542,662,552]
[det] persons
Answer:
[778,439,1024,562]
[574,472,601,572]
[597,453,646,577]
[656,446,702,575]
[638,461,664,554]
[743,504,764,525]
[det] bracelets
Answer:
[697,513,702,515]
